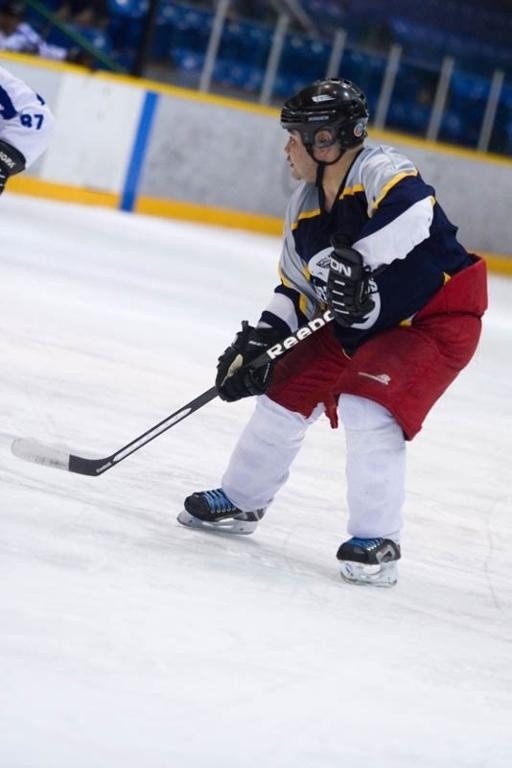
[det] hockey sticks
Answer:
[12,308,333,477]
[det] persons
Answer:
[173,80,486,586]
[0,2,102,193]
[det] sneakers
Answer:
[336,538,401,564]
[184,488,267,522]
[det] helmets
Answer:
[280,77,369,148]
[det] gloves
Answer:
[215,321,285,403]
[327,234,375,328]
